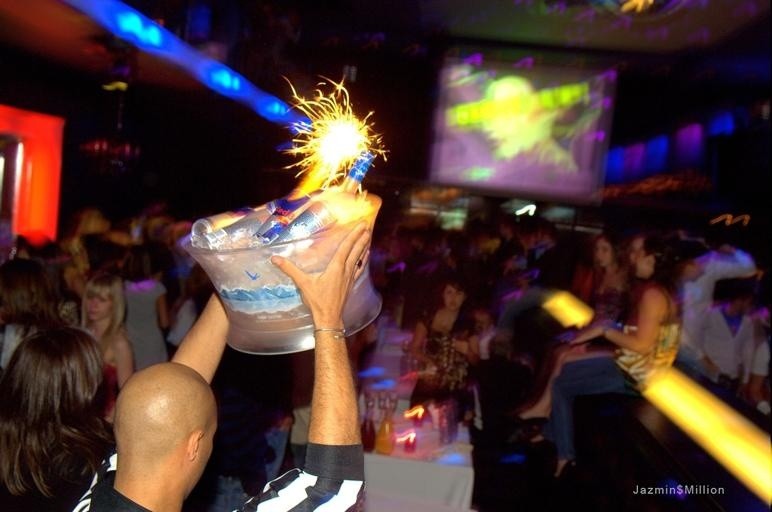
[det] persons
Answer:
[0,201,772,512]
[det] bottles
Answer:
[191,151,375,246]
[360,402,395,456]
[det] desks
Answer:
[352,314,478,510]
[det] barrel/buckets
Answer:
[184,191,383,354]
